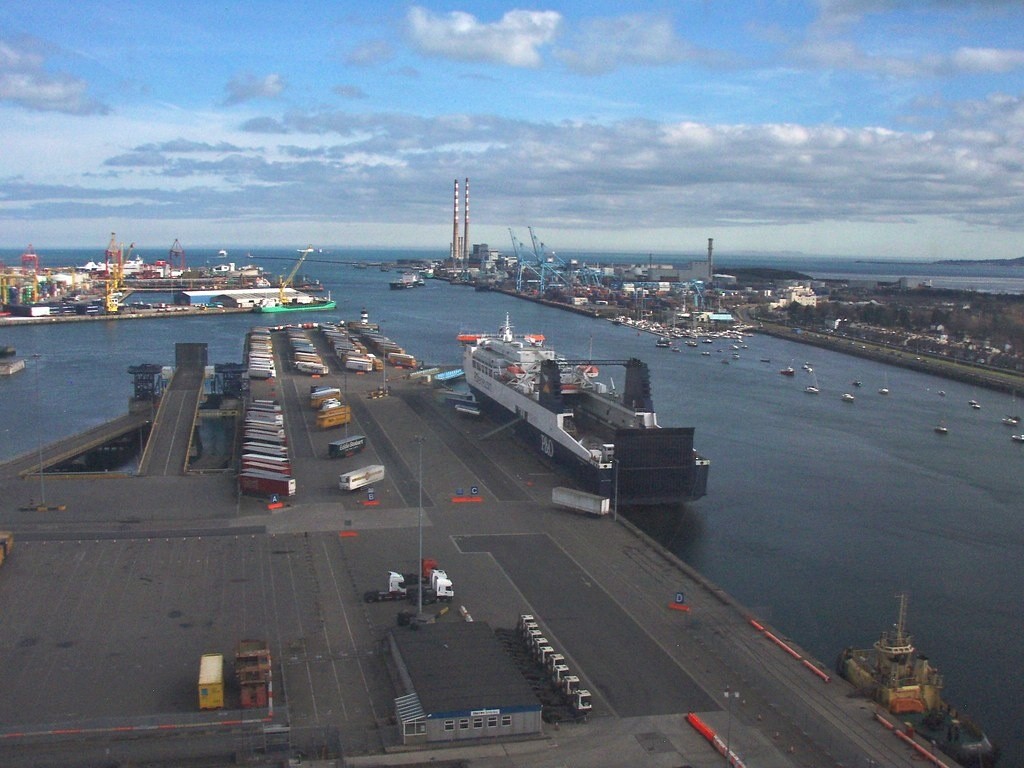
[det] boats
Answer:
[390,271,426,289]
[458,312,711,506]
[838,591,996,765]
[610,316,1023,444]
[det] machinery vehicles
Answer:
[280,245,315,303]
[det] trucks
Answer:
[197,638,269,711]
[495,613,592,725]
[365,556,454,606]
